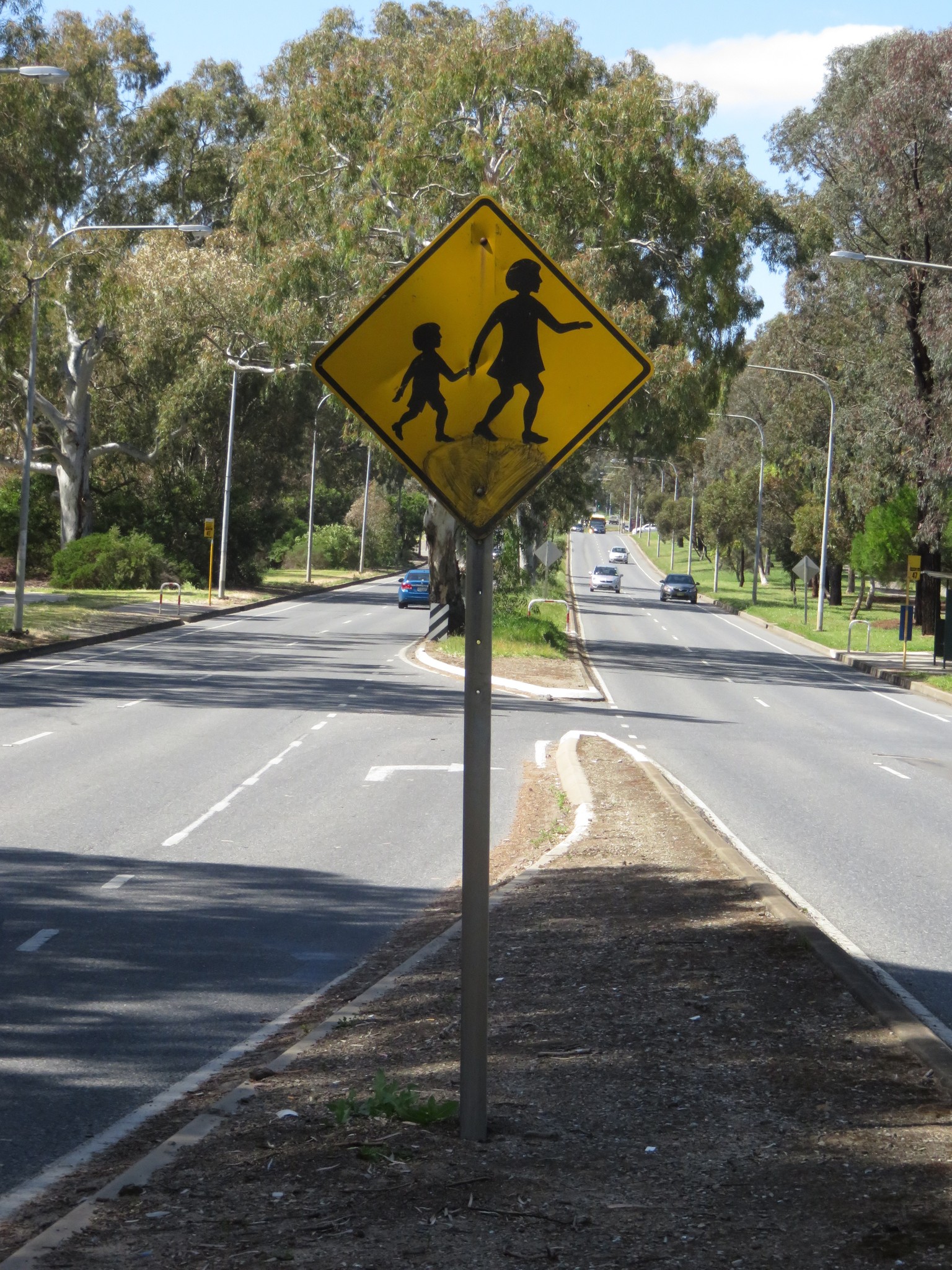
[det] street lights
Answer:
[6,223,212,637]
[596,452,678,570]
[216,340,377,598]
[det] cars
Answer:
[659,573,700,604]
[607,546,630,565]
[571,507,607,534]
[631,524,659,534]
[397,570,431,609]
[588,565,624,594]
[492,546,503,560]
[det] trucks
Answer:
[609,515,620,525]
